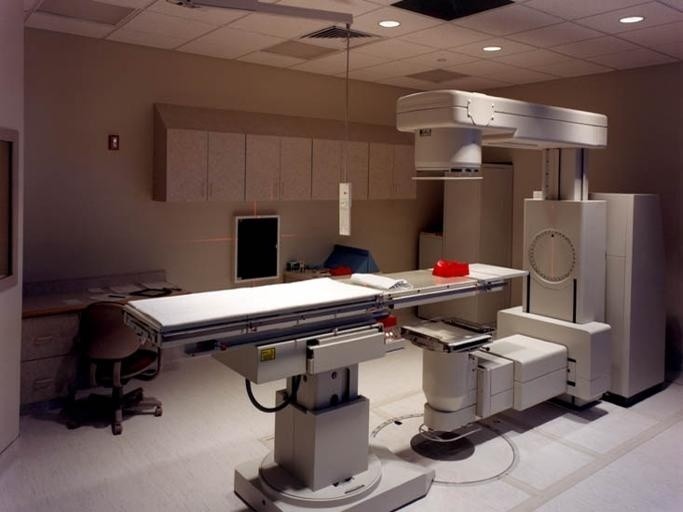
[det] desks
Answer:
[21,280,185,414]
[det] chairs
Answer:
[56,301,162,435]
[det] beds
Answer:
[123,263,529,512]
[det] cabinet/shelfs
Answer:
[153,101,415,201]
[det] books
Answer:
[85,279,182,304]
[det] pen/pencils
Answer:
[109,295,125,298]
